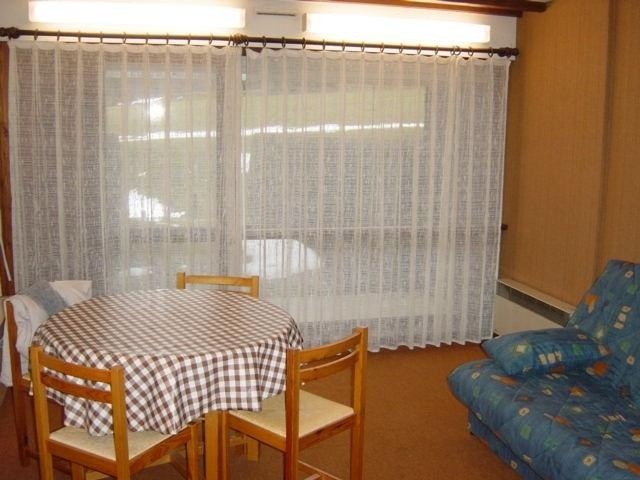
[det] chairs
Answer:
[3,279,112,479]
[28,345,199,480]
[221,325,374,480]
[173,270,266,462]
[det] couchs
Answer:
[444,255,640,478]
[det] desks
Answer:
[30,289,310,480]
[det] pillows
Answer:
[478,323,607,379]
